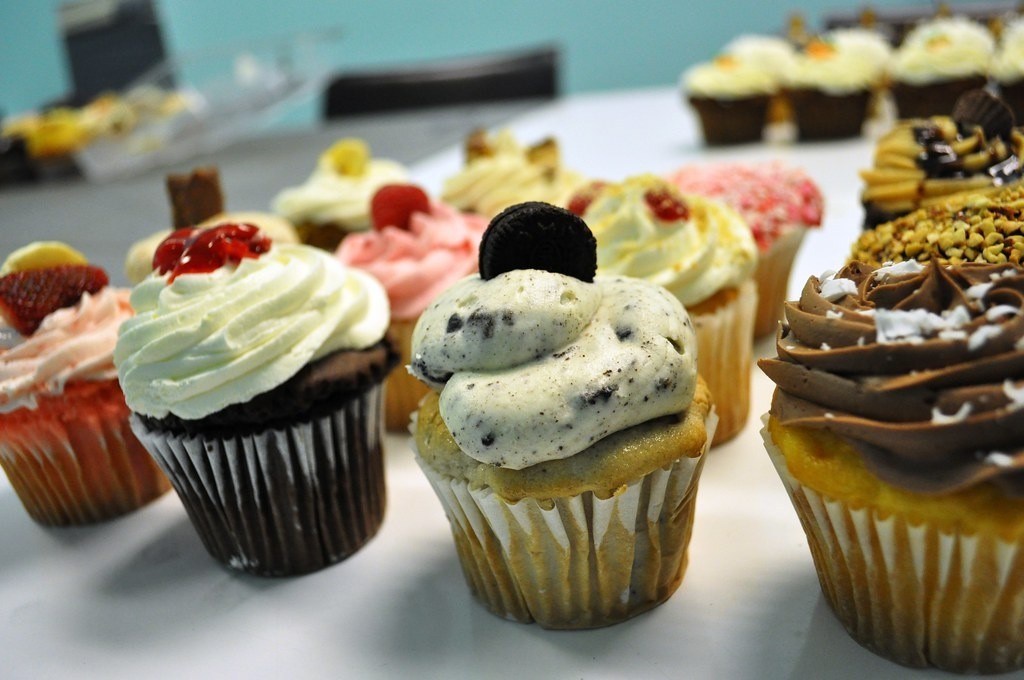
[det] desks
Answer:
[0,85,1024,680]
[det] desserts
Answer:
[0,127,824,629]
[684,6,1024,145]
[755,92,1024,674]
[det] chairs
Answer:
[319,42,563,123]
